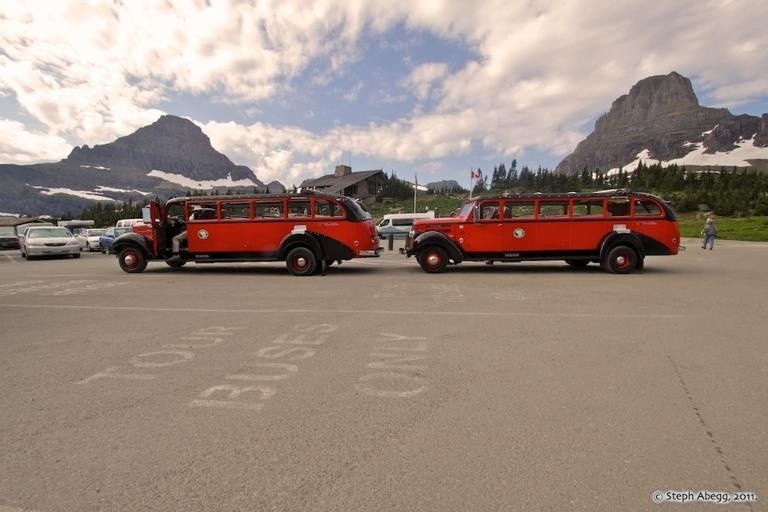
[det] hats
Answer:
[192,205,202,210]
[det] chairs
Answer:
[491,205,511,219]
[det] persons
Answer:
[169,209,196,258]
[701,217,718,250]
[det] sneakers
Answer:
[168,255,180,261]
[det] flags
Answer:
[471,171,481,179]
